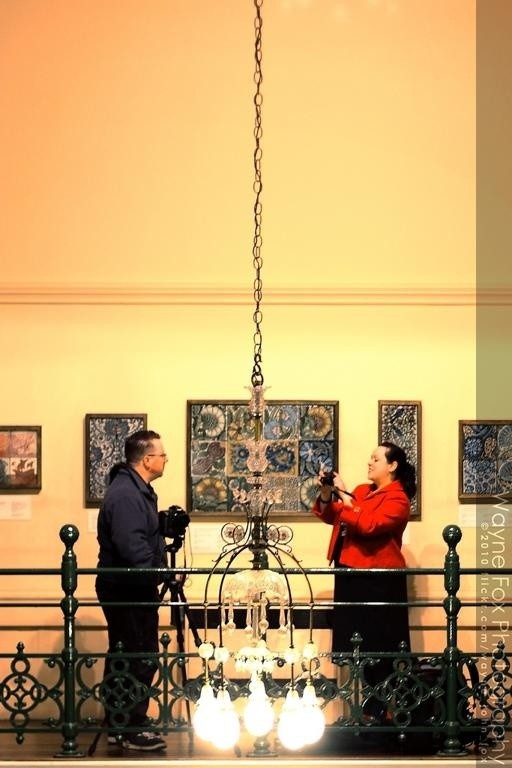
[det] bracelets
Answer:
[320,497,330,505]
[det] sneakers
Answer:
[107,728,167,750]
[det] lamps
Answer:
[189,0,327,752]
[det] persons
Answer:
[93,430,190,750]
[311,442,416,735]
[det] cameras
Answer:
[320,471,335,486]
[158,504,191,538]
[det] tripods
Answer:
[87,545,248,758]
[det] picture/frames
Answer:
[0,399,512,524]
